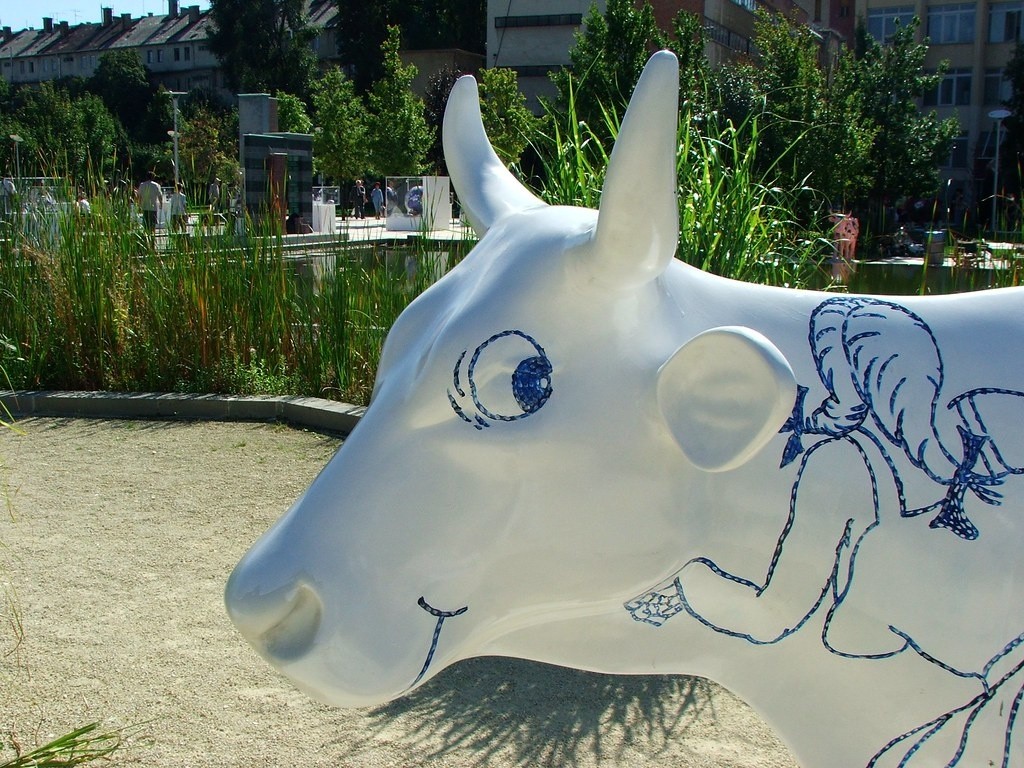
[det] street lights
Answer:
[988,108,1011,231]
[10,133,24,188]
[163,92,190,194]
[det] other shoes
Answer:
[375,212,380,219]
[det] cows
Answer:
[221,45,1023,768]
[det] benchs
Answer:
[955,240,989,268]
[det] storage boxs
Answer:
[312,186,340,205]
[385,175,451,230]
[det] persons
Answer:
[136,172,162,234]
[76,192,90,214]
[171,183,188,233]
[371,182,383,220]
[385,181,397,216]
[0,175,15,222]
[209,178,221,197]
[349,179,368,219]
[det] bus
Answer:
[6,177,85,193]
